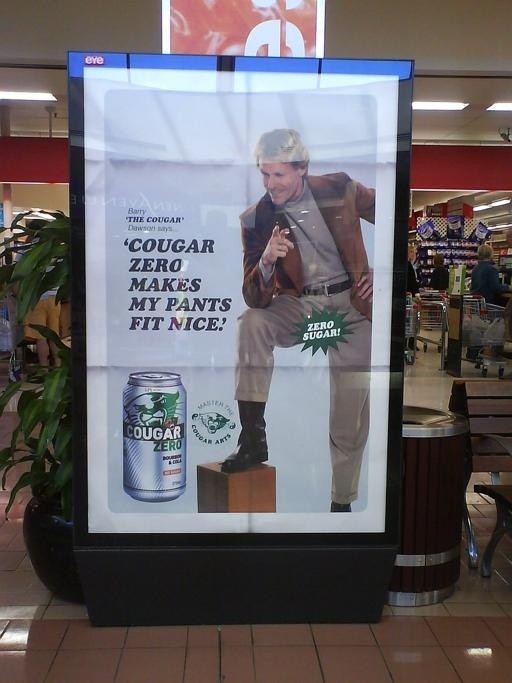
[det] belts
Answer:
[302,279,352,296]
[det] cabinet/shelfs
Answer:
[417,238,481,289]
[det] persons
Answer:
[221,130,375,512]
[470,244,512,305]
[406,261,420,297]
[431,254,449,290]
[13,219,62,364]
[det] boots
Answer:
[330,502,351,512]
[222,401,268,473]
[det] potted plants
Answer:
[0,204,96,605]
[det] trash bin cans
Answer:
[387,405,469,606]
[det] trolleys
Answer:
[404,291,416,365]
[461,293,509,378]
[416,285,449,331]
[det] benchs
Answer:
[446,378,512,578]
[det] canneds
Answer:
[122,369,187,502]
